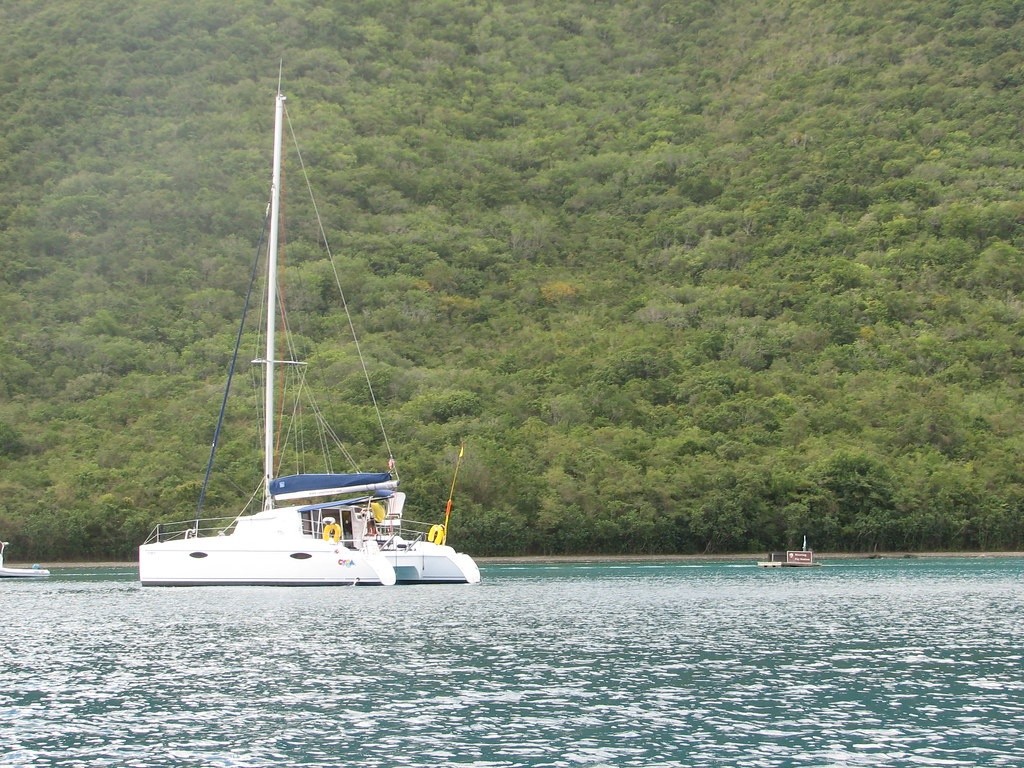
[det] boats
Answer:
[0,542,50,578]
[138,58,481,588]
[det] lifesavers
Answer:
[322,523,342,542]
[427,524,444,544]
